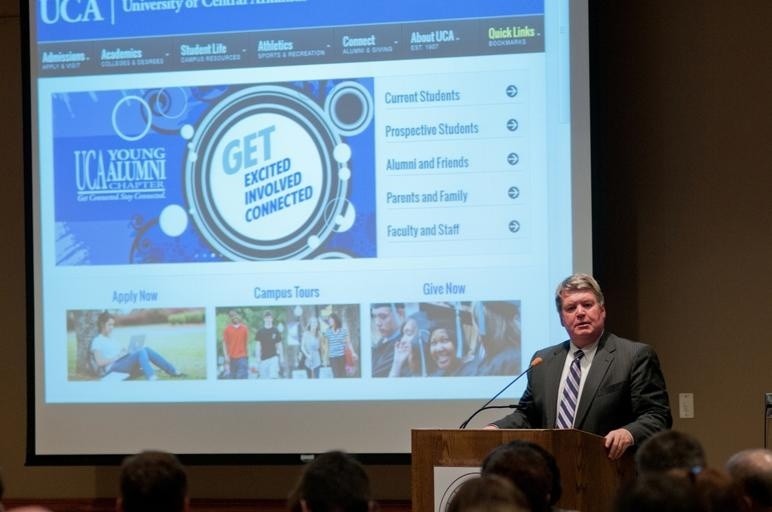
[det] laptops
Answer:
[114,333,146,362]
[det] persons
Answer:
[726,446,772,511]
[222,311,350,379]
[371,302,520,377]
[439,470,530,511]
[634,427,708,472]
[115,447,191,512]
[293,450,376,511]
[478,439,564,511]
[483,271,673,460]
[620,464,754,511]
[91,312,185,381]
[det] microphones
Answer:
[463,357,542,431]
[459,405,522,429]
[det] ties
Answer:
[555,350,585,430]
